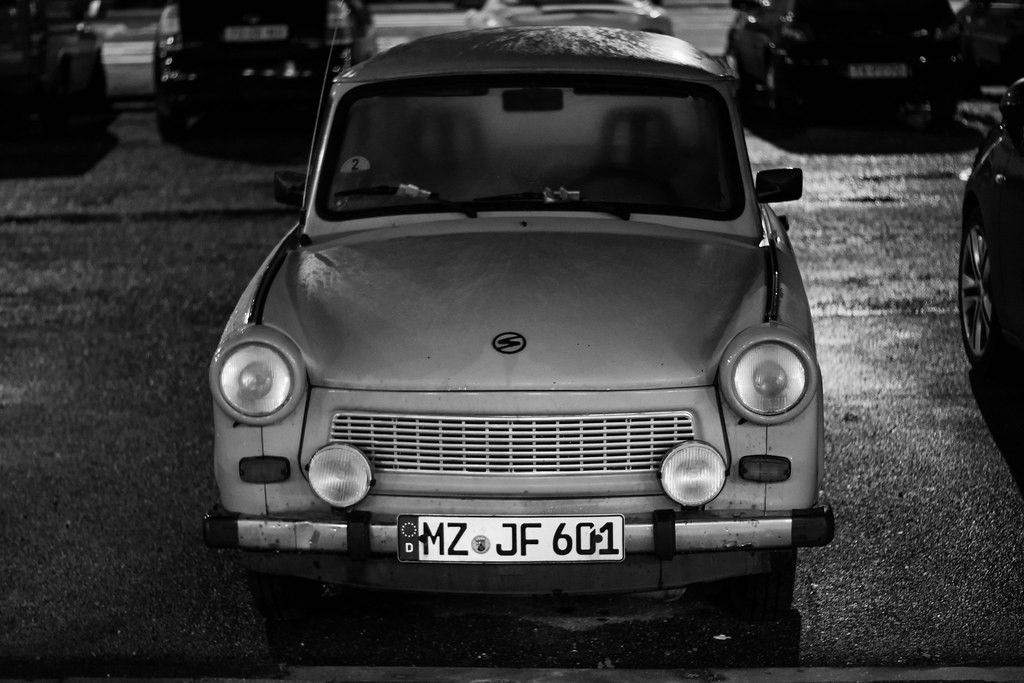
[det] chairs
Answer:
[579,106,697,207]
[390,107,499,200]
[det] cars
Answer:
[197,26,841,627]
[725,1,971,139]
[464,0,674,55]
[960,71,1024,395]
[954,0,1024,84]
[0,0,110,115]
[149,1,378,140]
